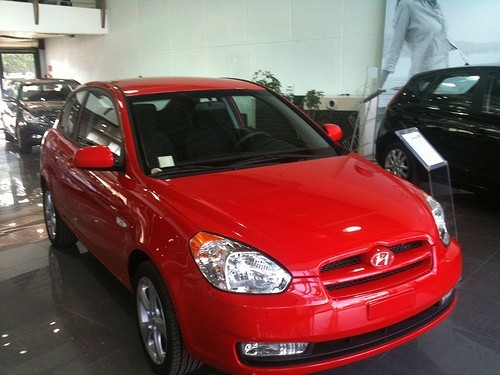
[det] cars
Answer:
[3,78,82,154]
[39,74,464,375]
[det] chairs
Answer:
[135,104,176,166]
[179,111,238,157]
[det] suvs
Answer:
[375,65,500,206]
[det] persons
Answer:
[378,0,459,96]
